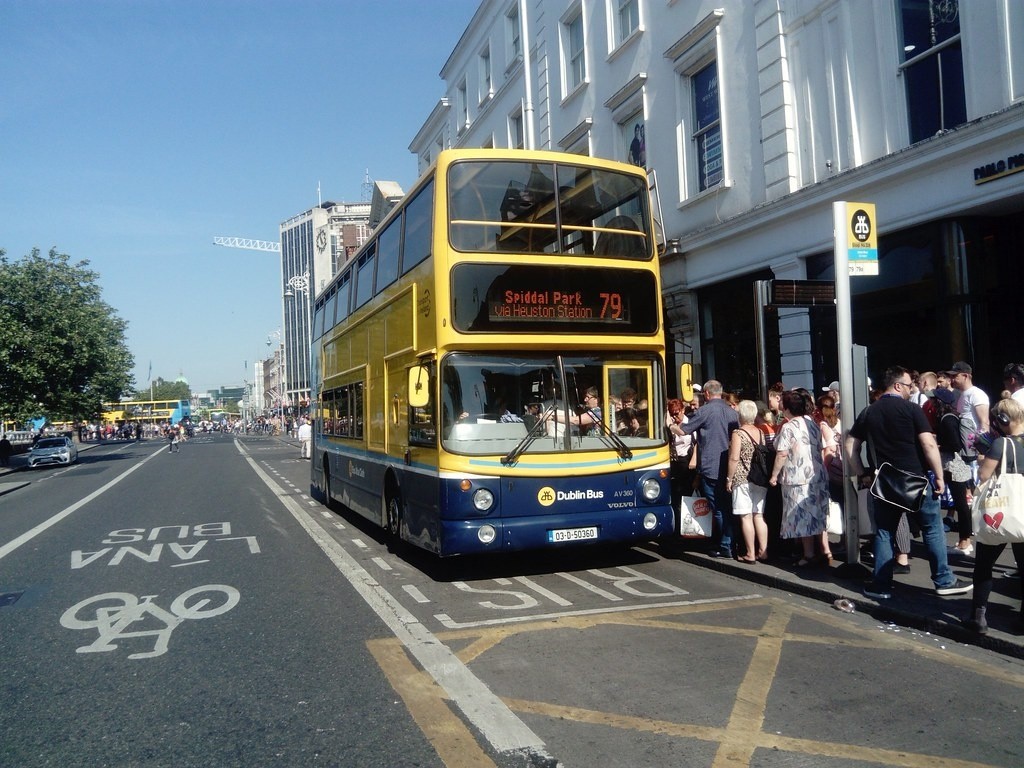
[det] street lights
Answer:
[265,331,284,431]
[285,274,312,367]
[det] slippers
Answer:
[755,550,769,563]
[735,555,756,563]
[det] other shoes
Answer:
[859,552,875,566]
[891,560,911,574]
[1003,569,1024,578]
[943,516,955,531]
[709,549,733,559]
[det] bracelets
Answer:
[551,415,554,420]
[726,476,732,482]
[669,424,675,428]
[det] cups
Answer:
[834,599,856,612]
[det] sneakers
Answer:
[936,578,975,594]
[946,546,970,555]
[954,541,973,552]
[864,585,892,599]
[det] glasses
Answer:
[623,400,635,405]
[894,380,913,389]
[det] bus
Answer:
[189,408,242,421]
[305,147,698,566]
[84,399,192,424]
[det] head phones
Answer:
[994,404,1010,427]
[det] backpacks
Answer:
[941,410,981,462]
[737,428,776,488]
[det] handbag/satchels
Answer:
[829,457,843,482]
[680,489,715,537]
[869,463,930,512]
[970,437,1024,545]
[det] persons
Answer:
[665,361,1024,634]
[21,419,194,454]
[197,412,309,439]
[32,434,43,446]
[323,416,363,436]
[298,419,311,459]
[445,386,648,440]
[629,123,645,168]
[0,434,12,467]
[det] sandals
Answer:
[817,554,834,564]
[793,555,818,569]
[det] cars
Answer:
[26,437,80,467]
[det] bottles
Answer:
[776,411,784,425]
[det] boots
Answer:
[963,607,988,633]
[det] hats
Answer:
[945,361,972,375]
[933,388,955,404]
[822,381,839,391]
[867,376,873,392]
